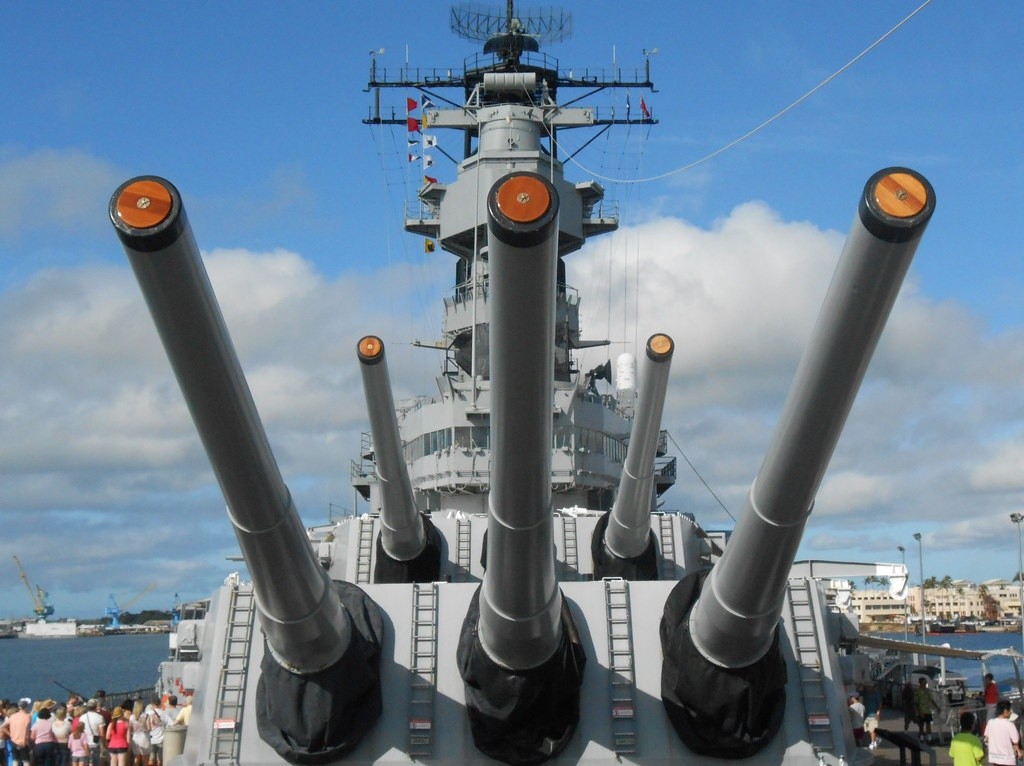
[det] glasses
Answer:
[922,682,927,684]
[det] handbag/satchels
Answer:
[94,735,100,743]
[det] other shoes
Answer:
[868,743,874,751]
[927,739,935,745]
[877,738,882,745]
[921,739,925,743]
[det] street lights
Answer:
[1011,512,1024,638]
[898,545,908,643]
[913,533,928,667]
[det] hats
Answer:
[97,690,105,697]
[187,696,193,704]
[113,706,125,718]
[863,681,871,686]
[40,699,56,709]
[17,698,31,709]
[904,682,913,688]
[849,691,860,698]
[86,698,98,707]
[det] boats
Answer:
[860,633,1024,745]
[915,618,957,633]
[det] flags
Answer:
[424,238,435,253]
[640,97,649,117]
[407,94,438,185]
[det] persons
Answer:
[948,712,984,766]
[1,689,193,766]
[983,700,1024,766]
[976,674,999,725]
[848,680,882,751]
[902,678,941,745]
